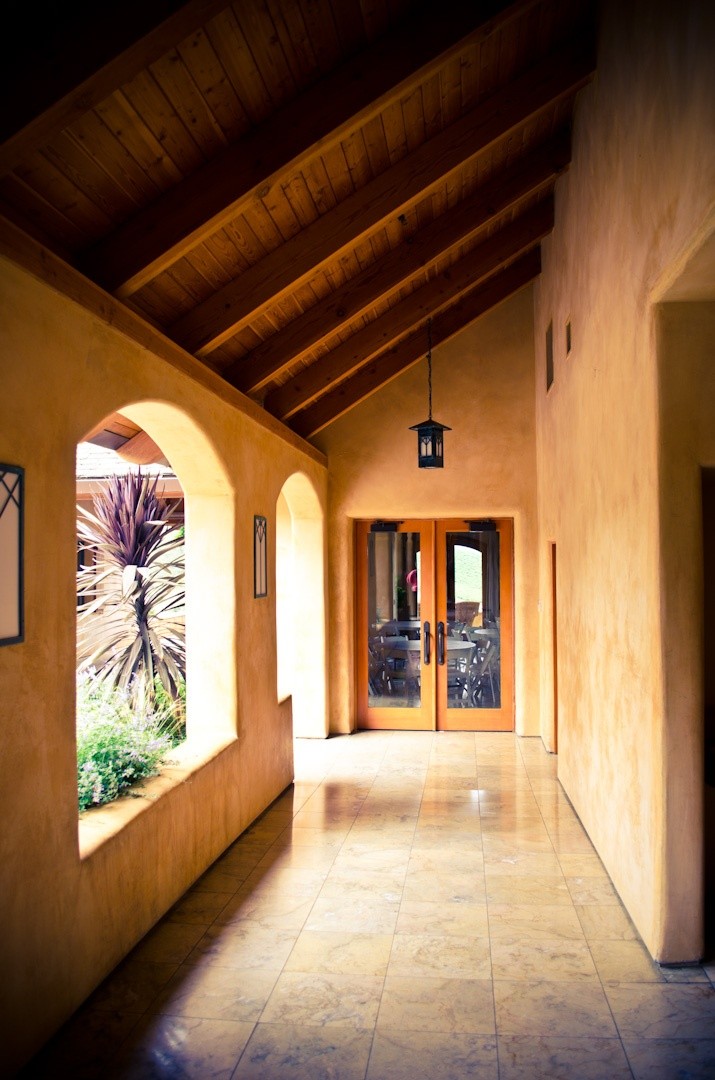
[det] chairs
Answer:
[367,610,500,709]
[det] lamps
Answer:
[409,317,453,470]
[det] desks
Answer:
[376,639,476,707]
[457,627,500,703]
[383,620,451,639]
